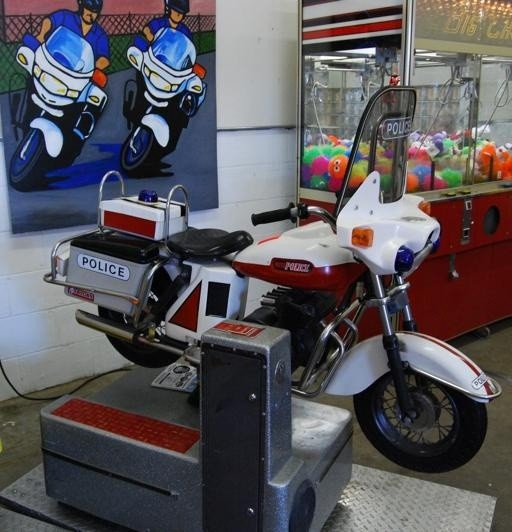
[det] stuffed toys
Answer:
[301,124,511,195]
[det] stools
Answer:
[168,228,253,256]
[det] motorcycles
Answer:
[5,25,109,191]
[43,83,501,473]
[122,26,207,178]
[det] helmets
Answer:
[164,0,190,15]
[78,0,103,15]
